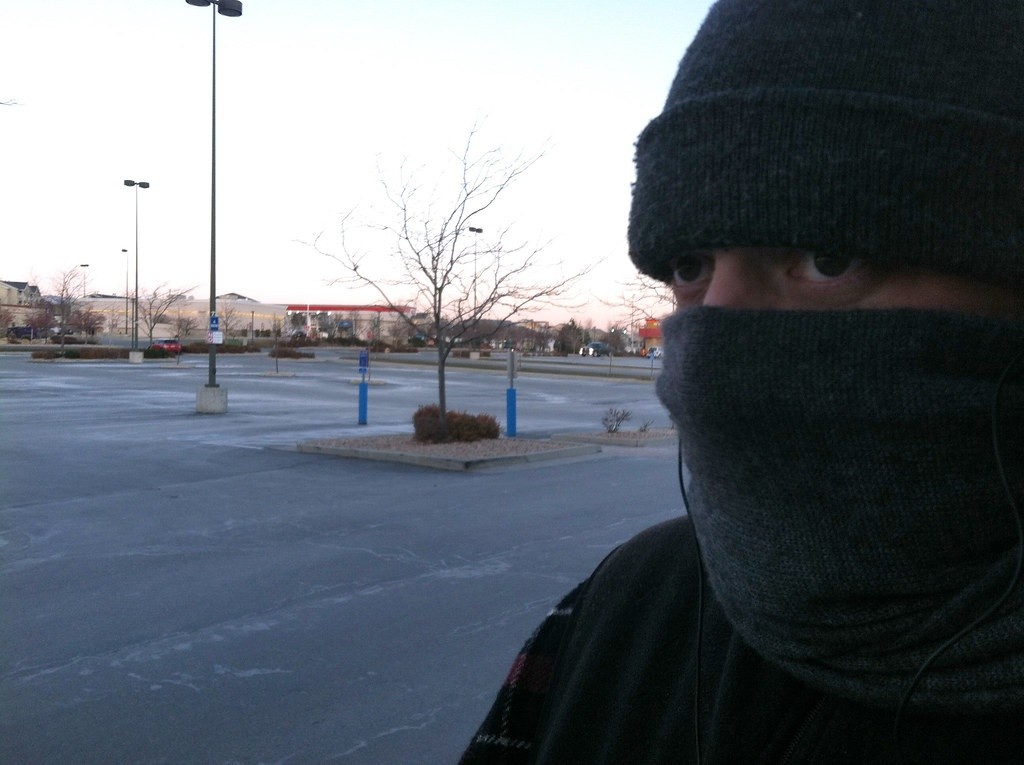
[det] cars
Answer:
[646,346,664,359]
[579,342,613,357]
[147,339,182,354]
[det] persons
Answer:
[454,0,1024,765]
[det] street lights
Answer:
[125,180,150,363]
[80,263,89,299]
[122,249,129,336]
[186,0,244,414]
[469,226,483,347]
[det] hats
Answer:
[628,1,1024,289]
